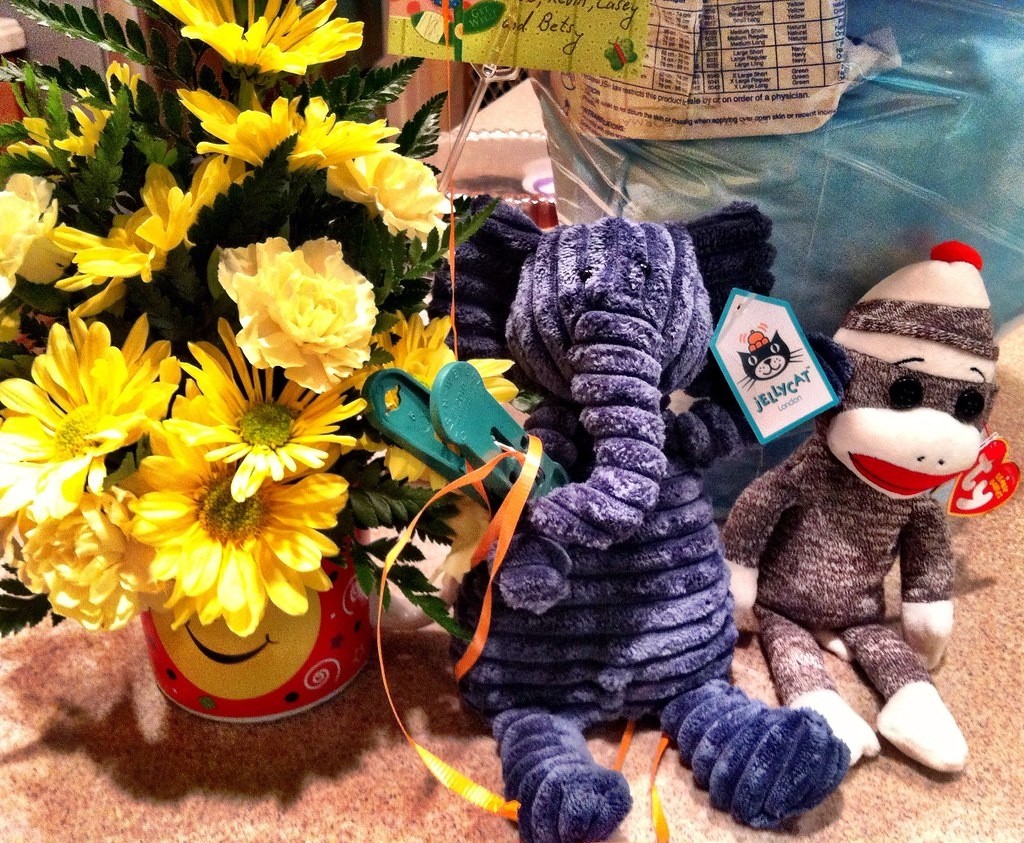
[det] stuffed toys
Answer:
[724,239,1000,777]
[429,192,851,843]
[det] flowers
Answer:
[0,0,520,642]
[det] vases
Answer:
[141,526,377,726]
